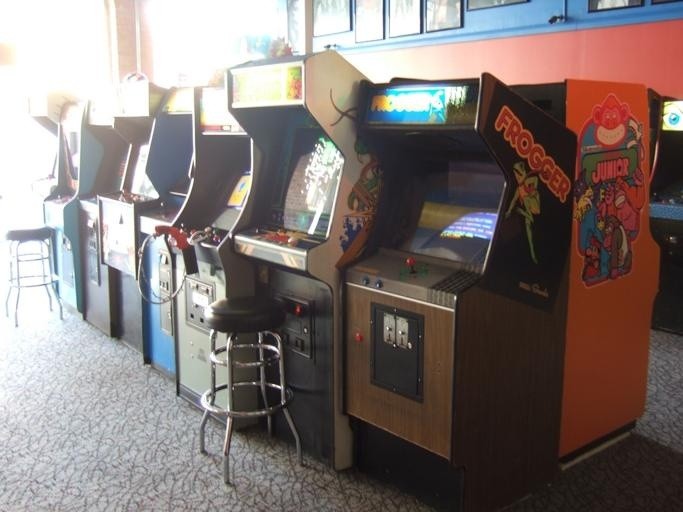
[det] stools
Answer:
[5,227,63,327]
[199,294,303,483]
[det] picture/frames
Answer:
[424,0,645,33]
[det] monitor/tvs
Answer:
[263,144,343,238]
[64,127,251,231]
[403,183,500,271]
